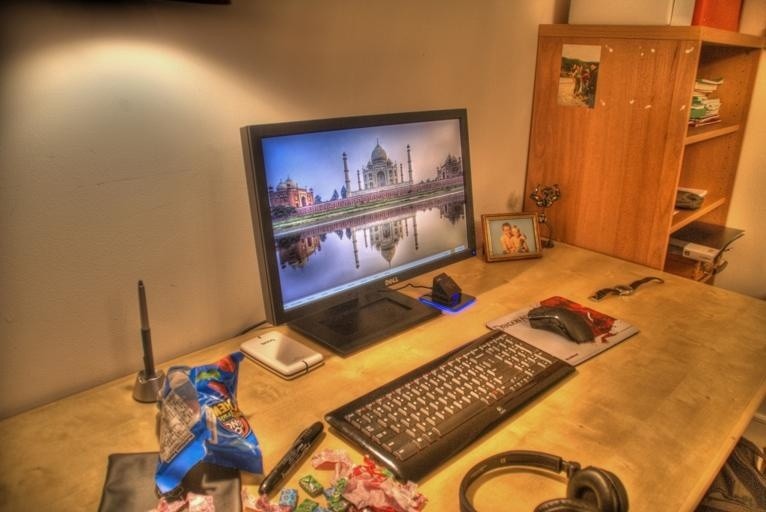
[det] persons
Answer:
[500,223,529,254]
[571,62,598,108]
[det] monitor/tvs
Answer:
[239,107,477,358]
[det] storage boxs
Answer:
[567,0,744,33]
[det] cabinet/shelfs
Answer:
[523,22,766,286]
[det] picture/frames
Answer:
[480,211,544,263]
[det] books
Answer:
[690,74,725,127]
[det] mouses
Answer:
[527,307,594,343]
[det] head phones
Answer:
[458,450,628,512]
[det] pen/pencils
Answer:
[259,421,324,495]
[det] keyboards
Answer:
[324,328,577,486]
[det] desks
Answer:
[0,233,766,512]
